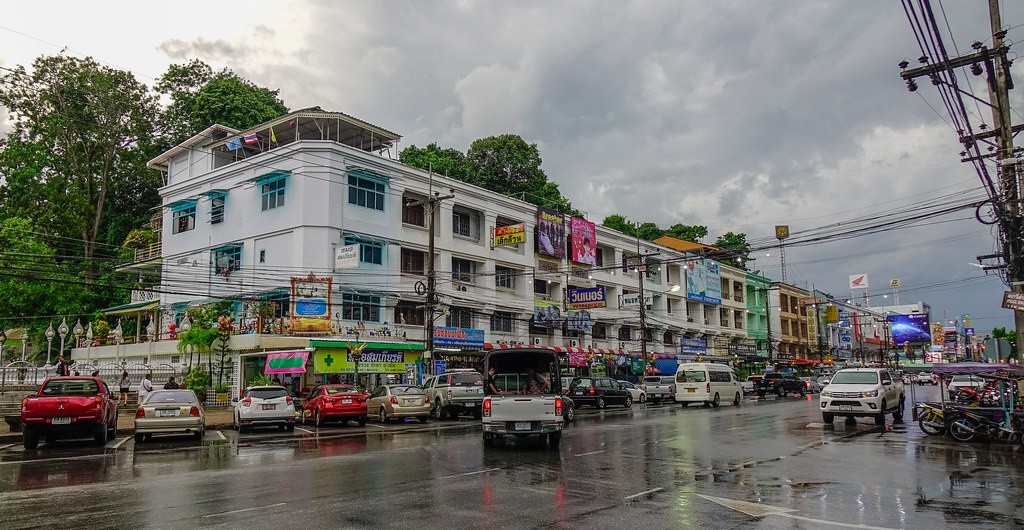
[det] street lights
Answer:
[0,329,9,366]
[45,321,57,368]
[147,314,157,367]
[638,283,681,374]
[85,321,94,374]
[20,326,30,360]
[113,319,124,378]
[180,311,192,373]
[58,317,70,361]
[72,317,84,370]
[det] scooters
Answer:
[912,378,1024,445]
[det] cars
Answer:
[742,372,832,397]
[641,376,678,404]
[558,377,572,393]
[366,384,432,424]
[232,385,297,432]
[617,379,647,404]
[300,384,369,426]
[917,372,939,384]
[902,374,919,385]
[947,375,986,400]
[133,389,207,443]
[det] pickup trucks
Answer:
[820,368,906,426]
[20,375,120,450]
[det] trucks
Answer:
[480,346,563,449]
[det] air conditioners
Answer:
[723,309,731,316]
[459,284,467,292]
[735,311,745,318]
[618,342,625,349]
[570,339,579,348]
[543,296,553,301]
[533,336,543,346]
[734,284,742,291]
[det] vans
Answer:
[674,362,745,408]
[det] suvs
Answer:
[567,375,634,409]
[416,367,486,421]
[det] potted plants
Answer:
[215,383,229,401]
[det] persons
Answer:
[522,365,548,395]
[288,376,293,383]
[138,374,152,406]
[291,377,300,396]
[55,357,77,376]
[16,362,27,384]
[688,258,705,295]
[400,312,406,323]
[220,268,230,277]
[540,216,563,247]
[271,374,281,385]
[245,324,253,334]
[74,372,79,375]
[357,320,364,331]
[577,238,595,265]
[486,366,500,395]
[163,376,179,389]
[117,372,131,406]
[91,370,100,377]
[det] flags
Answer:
[224,136,242,151]
[244,131,257,144]
[270,125,276,142]
